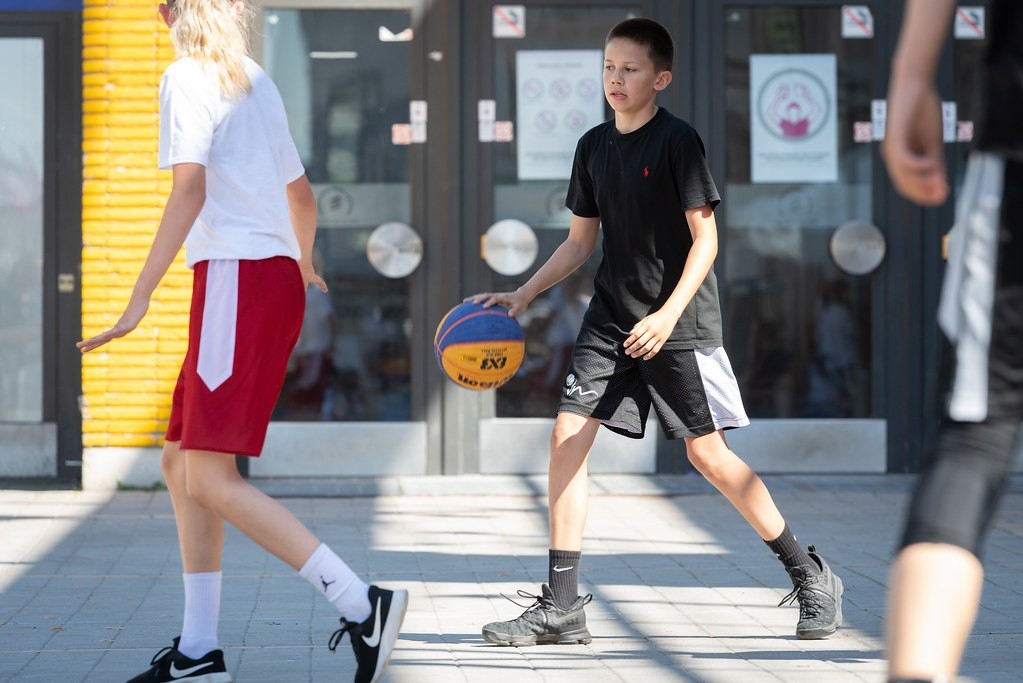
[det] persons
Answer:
[76,0,408,683]
[882,0,1023,683]
[462,18,843,646]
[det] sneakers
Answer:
[778,544,844,640]
[482,584,593,646]
[328,585,409,683]
[126,636,233,683]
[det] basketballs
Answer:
[433,299,527,392]
